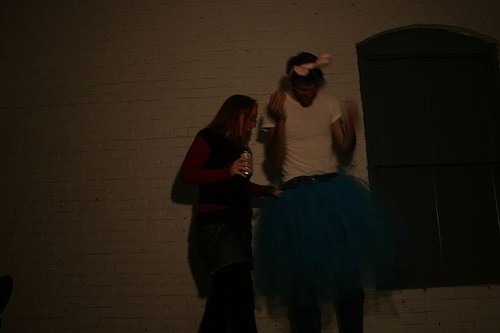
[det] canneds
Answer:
[238,146,253,178]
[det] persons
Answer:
[178,92,286,332]
[261,51,369,332]
